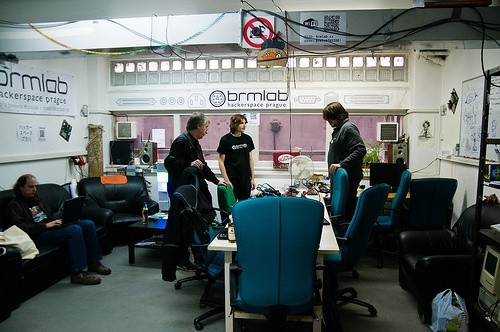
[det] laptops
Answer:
[48,195,85,229]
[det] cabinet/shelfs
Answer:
[472,66,500,332]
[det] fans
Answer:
[288,155,314,189]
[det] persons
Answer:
[162,113,227,281]
[323,102,366,221]
[5,174,111,285]
[217,115,256,202]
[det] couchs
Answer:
[0,184,113,323]
[397,200,500,325]
[77,175,158,246]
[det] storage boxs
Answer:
[272,151,301,169]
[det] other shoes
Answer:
[71,271,102,285]
[88,263,112,275]
[175,257,198,271]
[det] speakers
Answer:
[140,142,157,166]
[388,143,407,165]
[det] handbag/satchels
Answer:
[432,289,468,332]
[0,224,40,259]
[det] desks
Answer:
[208,195,339,332]
[128,213,168,265]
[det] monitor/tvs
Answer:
[480,244,500,295]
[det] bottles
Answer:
[228,206,236,242]
[142,204,148,225]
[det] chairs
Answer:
[172,168,458,332]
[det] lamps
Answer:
[257,31,288,67]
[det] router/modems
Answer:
[141,132,151,142]
